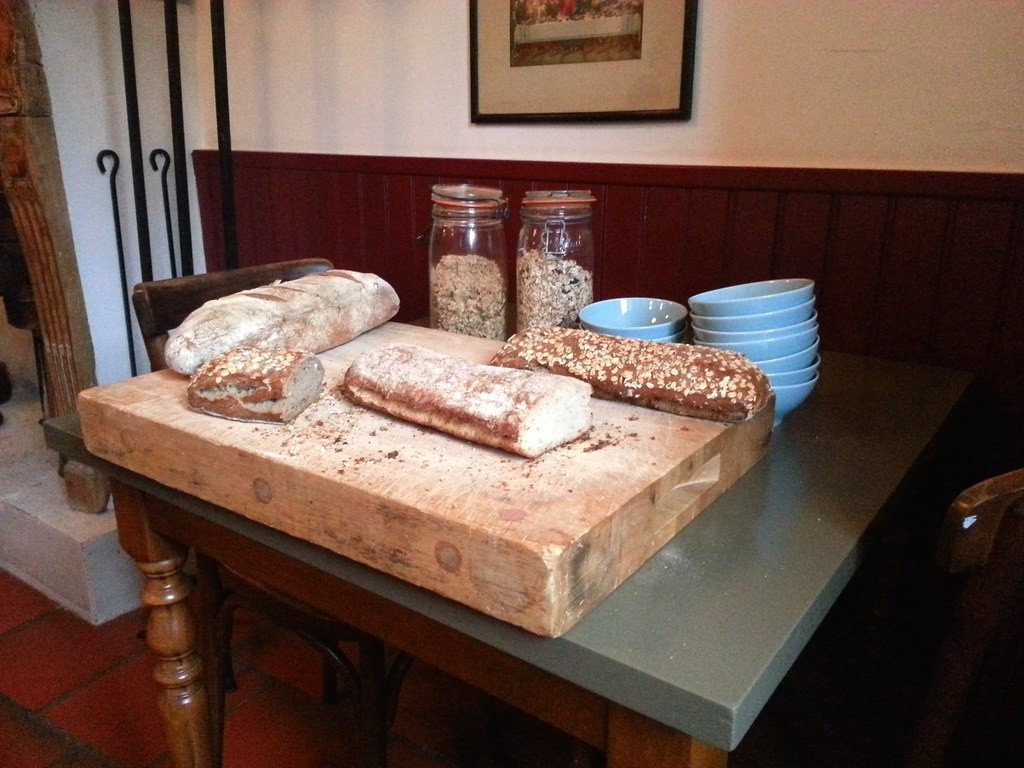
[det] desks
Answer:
[37,389,942,768]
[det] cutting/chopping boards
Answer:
[76,322,777,639]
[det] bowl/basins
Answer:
[578,278,821,428]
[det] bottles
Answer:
[429,184,510,343]
[515,189,596,330]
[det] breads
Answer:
[342,341,594,458]
[489,325,771,422]
[186,346,327,425]
[164,269,401,375]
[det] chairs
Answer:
[903,468,1024,768]
[131,257,419,768]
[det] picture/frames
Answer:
[469,0,698,123]
